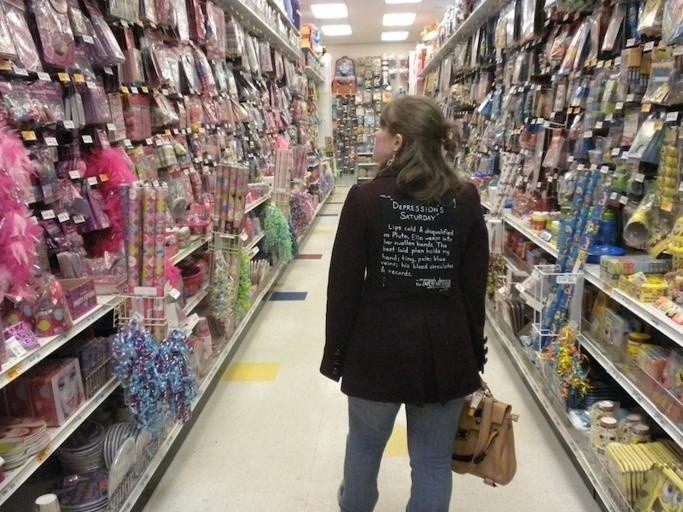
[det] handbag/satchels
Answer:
[450,382,519,487]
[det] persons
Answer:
[316,91,491,511]
[55,367,83,417]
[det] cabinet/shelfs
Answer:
[0,165,335,512]
[481,193,683,512]
